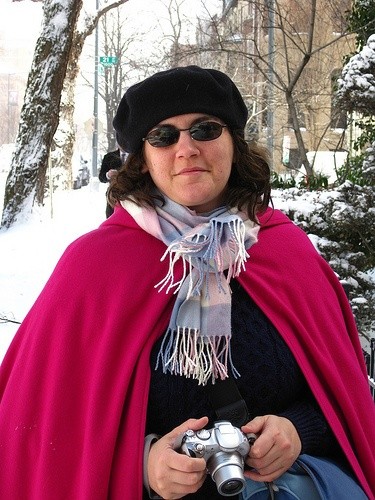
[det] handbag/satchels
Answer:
[206,372,369,500]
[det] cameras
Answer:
[178,420,258,496]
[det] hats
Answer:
[112,65,249,152]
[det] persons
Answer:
[98,145,130,218]
[0,65,375,500]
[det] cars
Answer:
[71,153,90,189]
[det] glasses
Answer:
[141,120,227,148]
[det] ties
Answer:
[122,154,125,162]
